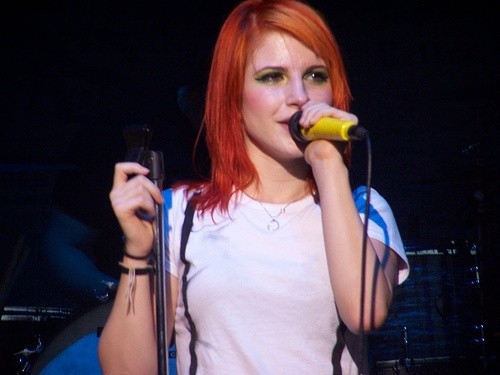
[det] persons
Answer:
[96,0,410,375]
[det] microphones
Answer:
[289,110,368,144]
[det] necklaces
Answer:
[241,181,313,232]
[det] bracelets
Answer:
[116,261,154,275]
[122,245,151,260]
